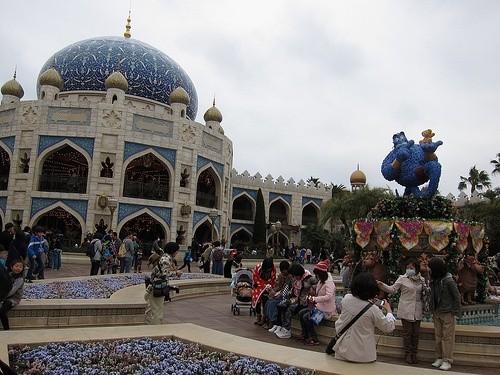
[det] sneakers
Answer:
[275,328,291,339]
[432,358,442,367]
[440,362,452,370]
[274,326,280,333]
[269,325,276,332]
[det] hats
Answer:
[148,253,162,269]
[314,259,330,272]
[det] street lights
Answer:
[107,198,118,231]
[209,209,219,243]
[275,220,281,256]
[271,224,275,248]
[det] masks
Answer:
[406,269,418,276]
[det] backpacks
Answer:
[213,247,223,261]
[117,238,130,258]
[86,239,101,257]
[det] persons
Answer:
[266,244,274,258]
[428,257,461,370]
[376,258,427,364]
[251,257,336,346]
[1,246,26,330]
[191,237,223,275]
[0,223,65,284]
[145,242,183,325]
[84,230,164,276]
[179,246,192,273]
[245,246,258,255]
[280,242,311,265]
[319,243,348,273]
[332,272,396,363]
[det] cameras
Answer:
[374,298,384,306]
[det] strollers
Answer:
[230,268,258,317]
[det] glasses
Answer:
[24,278,31,283]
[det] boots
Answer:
[259,315,266,326]
[254,314,261,324]
[262,320,276,330]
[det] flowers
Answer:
[350,196,489,303]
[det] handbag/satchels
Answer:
[326,334,339,355]
[152,282,168,297]
[421,278,429,303]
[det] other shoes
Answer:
[297,334,308,340]
[25,275,36,280]
[39,277,44,280]
[406,353,418,364]
[306,337,320,344]
